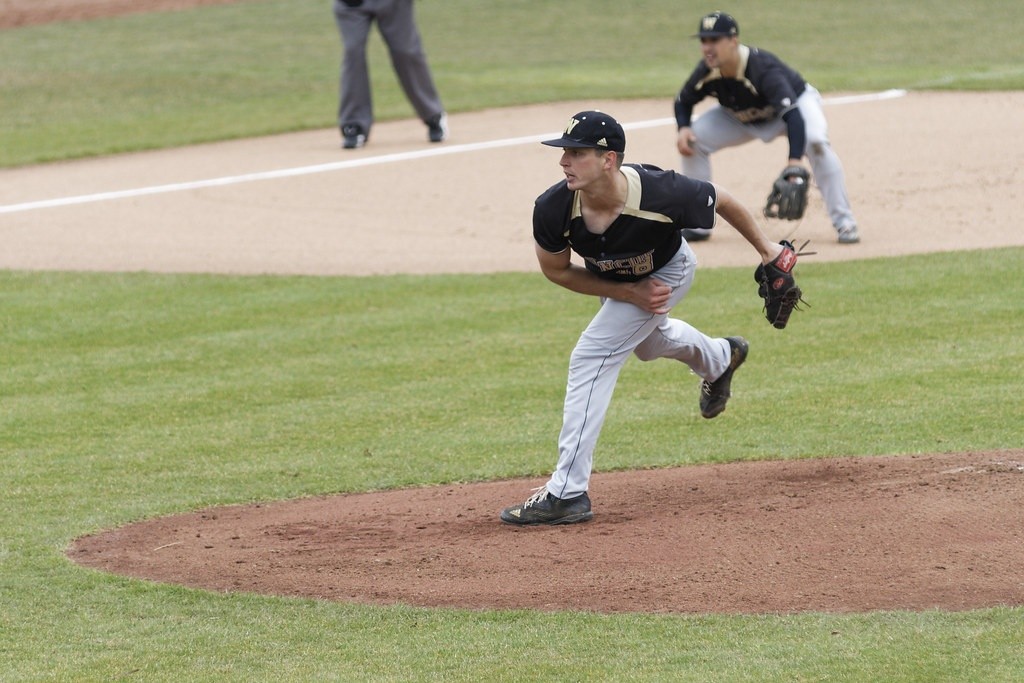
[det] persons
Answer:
[665,8,864,248]
[329,0,449,150]
[497,107,803,530]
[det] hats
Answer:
[541,109,626,153]
[689,10,738,38]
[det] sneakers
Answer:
[342,122,366,149]
[425,111,446,142]
[680,227,712,241]
[838,226,860,243]
[699,335,749,419]
[499,491,594,525]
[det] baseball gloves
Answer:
[762,164,812,220]
[753,239,817,329]
